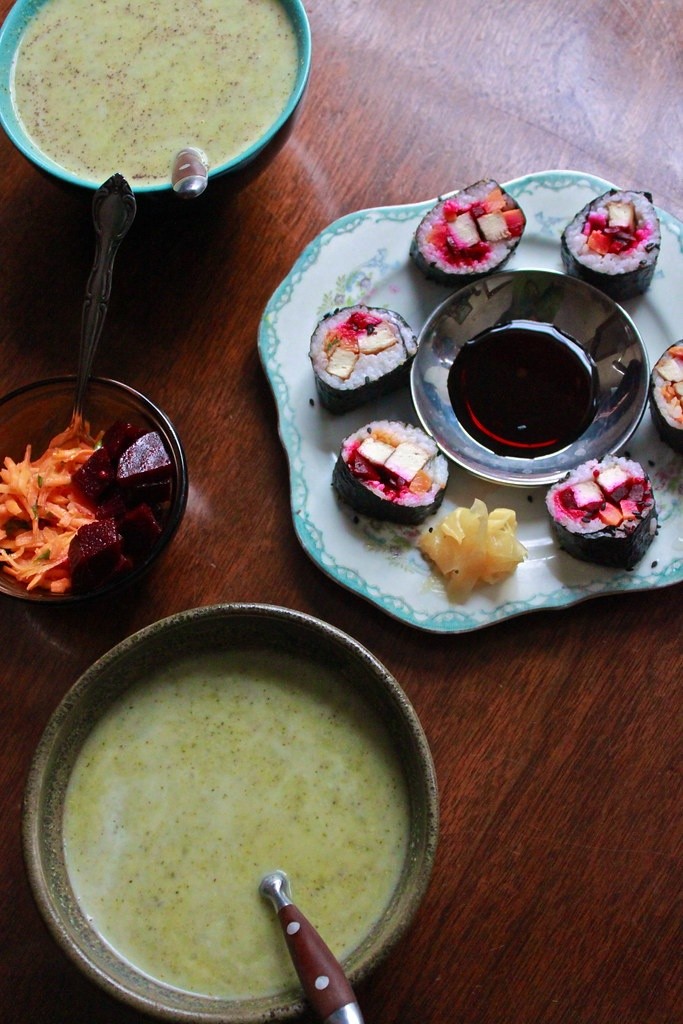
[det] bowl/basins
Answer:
[0,374,187,613]
[21,603,439,1024]
[407,269,649,487]
[0,1,311,204]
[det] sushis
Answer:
[310,179,683,570]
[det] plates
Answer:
[259,172,682,635]
